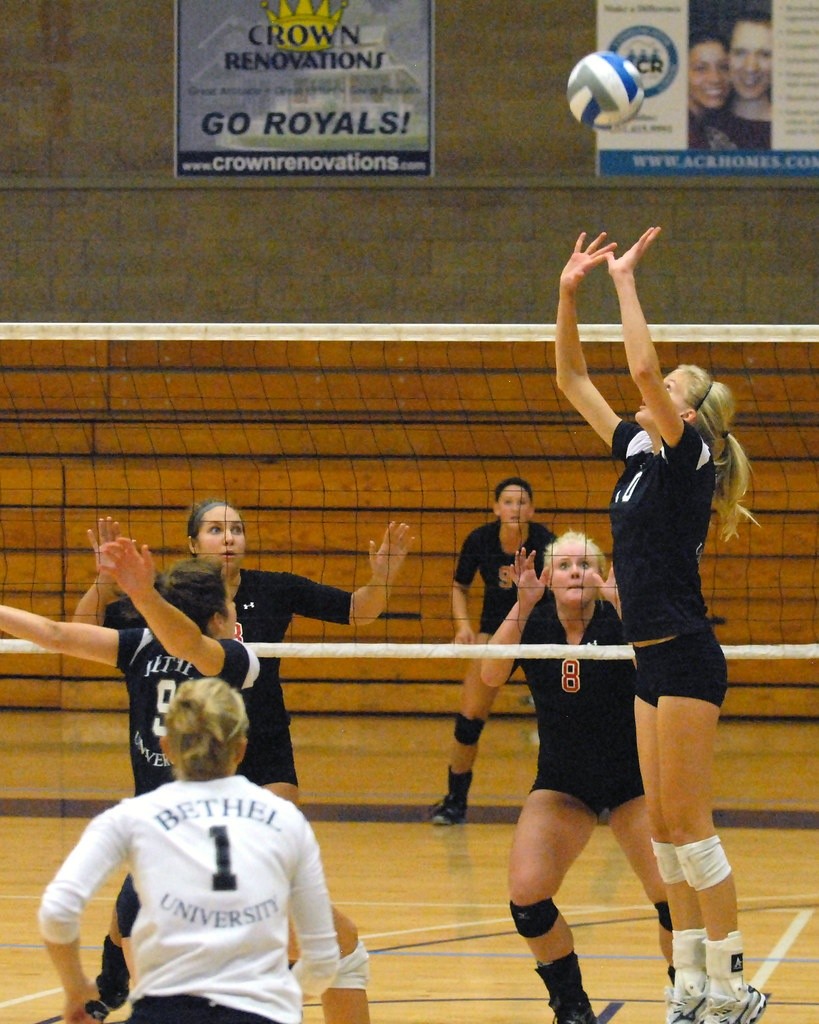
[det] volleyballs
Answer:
[565,50,645,131]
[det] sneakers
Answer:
[665,985,709,1024]
[699,984,767,1024]
[432,791,467,826]
[549,991,596,1024]
[85,976,128,1022]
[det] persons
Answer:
[553,225,777,1024]
[687,10,773,150]
[71,498,420,806]
[429,476,560,824]
[479,530,679,1024]
[0,540,370,1024]
